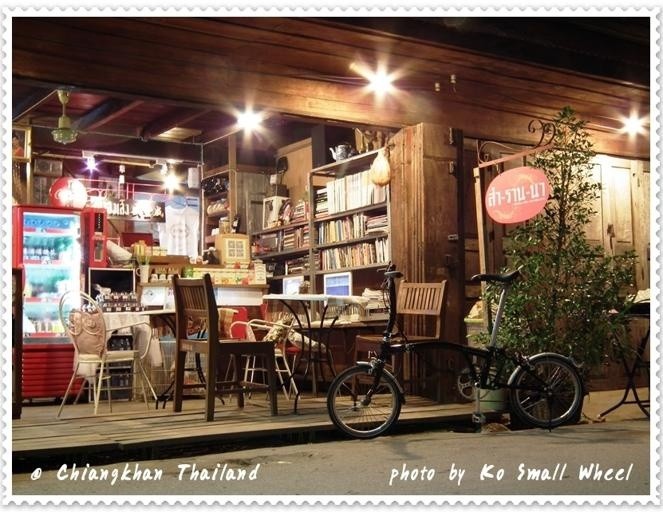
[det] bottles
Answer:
[118,371,129,387]
[23,235,73,262]
[118,338,131,351]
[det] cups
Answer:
[153,246,167,257]
[136,265,150,283]
[152,274,173,282]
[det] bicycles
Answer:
[327,265,584,439]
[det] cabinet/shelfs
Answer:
[251,146,398,397]
[200,166,276,262]
[9,146,206,263]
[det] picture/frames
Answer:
[12,124,31,163]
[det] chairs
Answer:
[55,289,159,418]
[218,306,330,401]
[169,274,281,423]
[351,280,448,405]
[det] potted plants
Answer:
[472,104,638,427]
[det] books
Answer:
[250,165,390,310]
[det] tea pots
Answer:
[329,141,354,162]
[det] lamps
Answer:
[50,86,79,145]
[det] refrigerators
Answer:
[13,205,108,404]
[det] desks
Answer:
[593,298,650,421]
[256,293,368,411]
